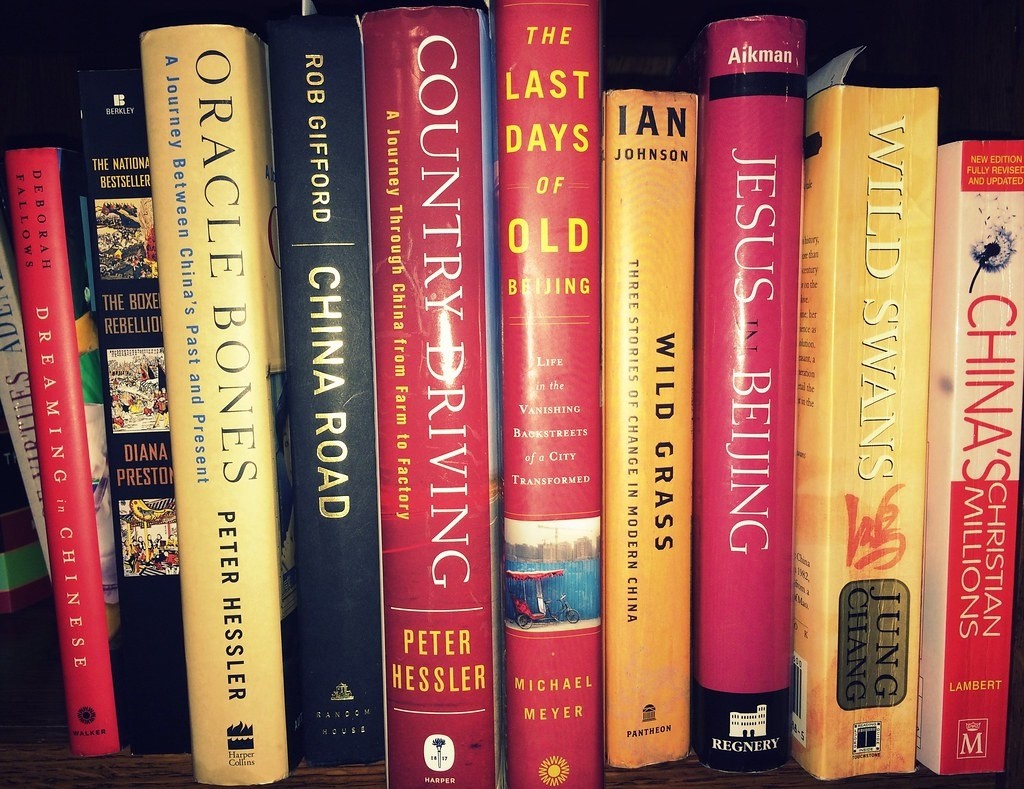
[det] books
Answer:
[0,0,1024,789]
